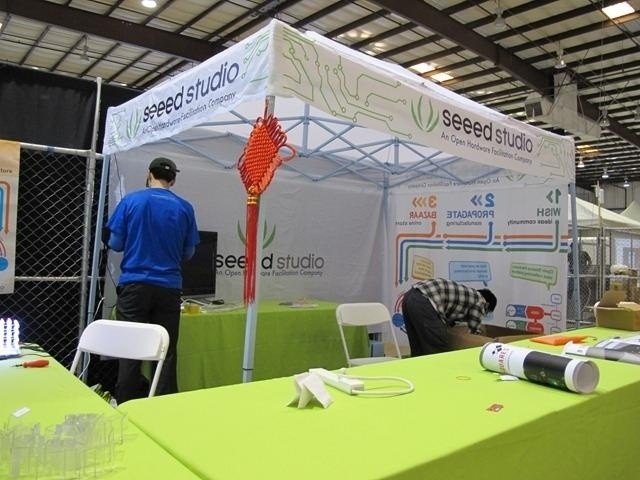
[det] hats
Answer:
[145,157,177,187]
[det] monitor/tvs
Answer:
[182,231,217,306]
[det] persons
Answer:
[402,277,497,358]
[105,159,200,403]
[566,241,592,310]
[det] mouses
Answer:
[213,300,224,305]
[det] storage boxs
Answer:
[585,291,640,331]
[448,324,540,350]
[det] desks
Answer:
[110,299,370,393]
[115,326,640,480]
[0,344,203,480]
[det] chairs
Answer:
[336,303,401,368]
[70,318,170,398]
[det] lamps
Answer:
[555,40,566,68]
[577,157,584,167]
[79,38,89,62]
[624,180,629,186]
[600,86,610,126]
[602,168,609,177]
[495,0,505,27]
